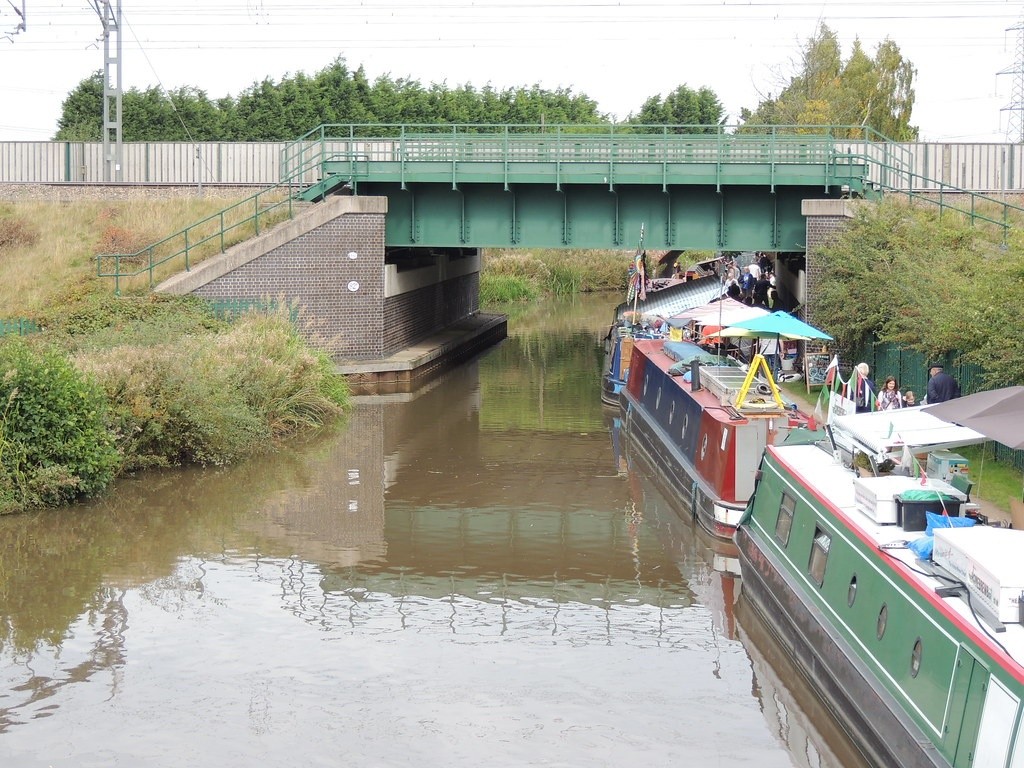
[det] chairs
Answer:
[977,512,1002,527]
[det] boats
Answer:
[730,426,1024,768]
[619,334,821,540]
[598,317,673,407]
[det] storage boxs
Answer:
[892,491,964,532]
[779,340,798,370]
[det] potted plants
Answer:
[854,449,896,478]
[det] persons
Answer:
[754,273,776,308]
[877,375,902,411]
[927,363,959,451]
[725,251,785,311]
[759,339,784,385]
[742,267,754,297]
[902,391,920,408]
[850,363,876,413]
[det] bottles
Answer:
[791,403,797,419]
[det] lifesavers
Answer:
[780,373,802,382]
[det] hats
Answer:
[927,362,943,370]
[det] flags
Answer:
[627,238,643,306]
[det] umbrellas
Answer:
[672,297,773,366]
[920,386,1024,450]
[708,327,812,379]
[725,310,834,384]
